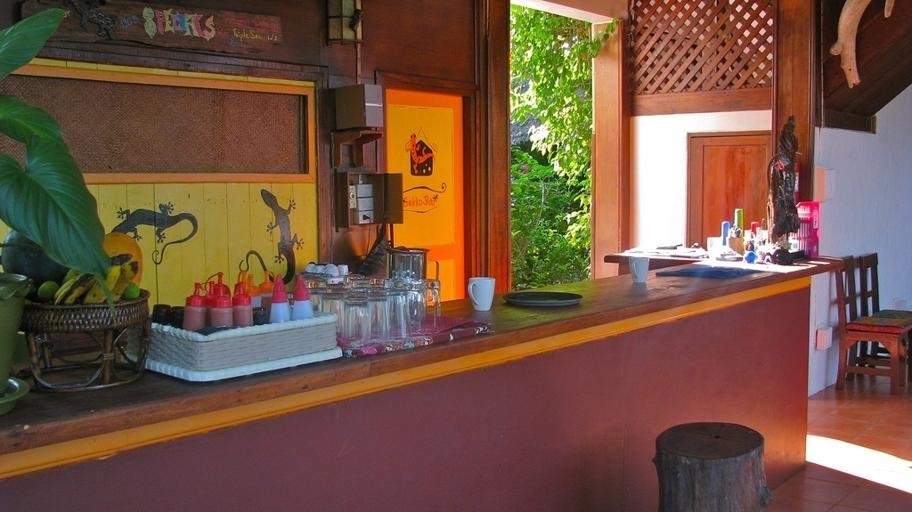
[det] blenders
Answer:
[382,243,442,282]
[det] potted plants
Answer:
[0,6,114,414]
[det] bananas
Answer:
[65,254,139,305]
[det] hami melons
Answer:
[103,232,142,286]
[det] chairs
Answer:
[836,253,912,395]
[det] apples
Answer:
[38,281,59,302]
[121,282,141,300]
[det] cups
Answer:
[299,271,448,344]
[466,276,498,312]
[626,254,652,285]
[304,261,351,277]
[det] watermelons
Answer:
[3,230,70,304]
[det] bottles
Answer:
[178,266,317,334]
